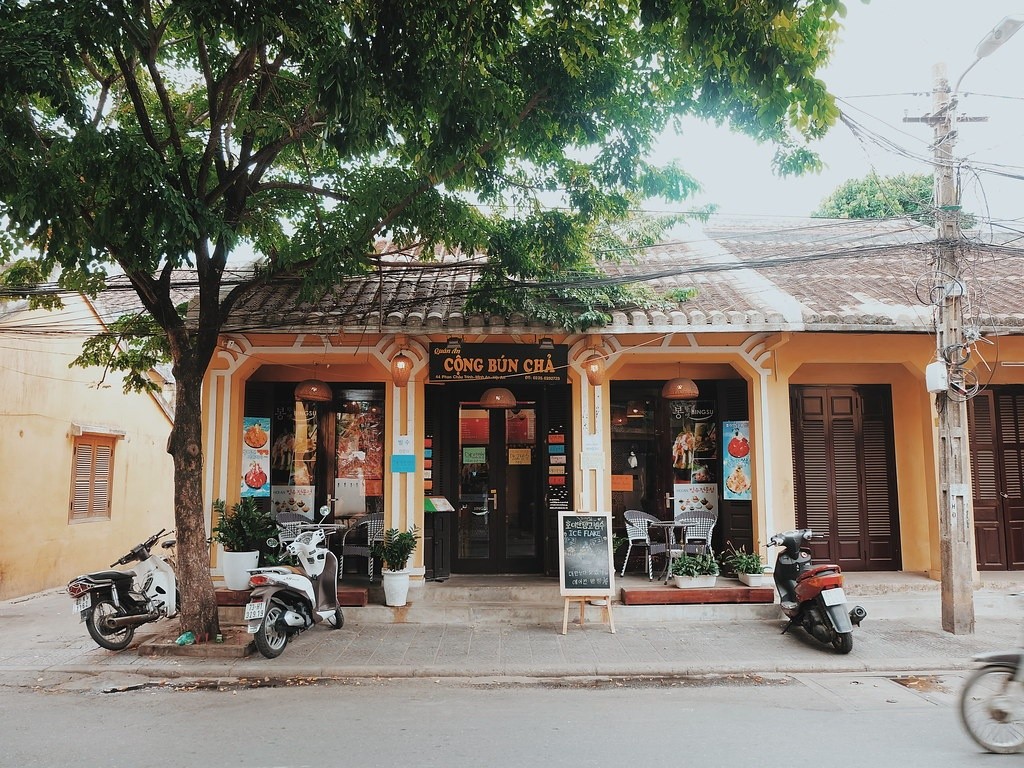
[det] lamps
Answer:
[294,366,332,402]
[481,388,517,407]
[446,338,462,349]
[539,339,556,349]
[392,348,412,387]
[586,346,604,386]
[614,363,699,425]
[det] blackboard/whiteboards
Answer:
[557,510,616,597]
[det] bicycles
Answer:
[160,540,179,619]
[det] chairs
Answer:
[273,511,385,581]
[622,509,717,581]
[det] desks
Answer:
[295,524,346,552]
[652,520,697,584]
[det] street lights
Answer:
[934,14,1024,635]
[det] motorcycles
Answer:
[244,506,345,660]
[761,529,866,655]
[960,653,1024,755]
[66,528,181,652]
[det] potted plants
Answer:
[209,496,277,591]
[724,541,774,586]
[371,524,422,606]
[668,551,719,589]
